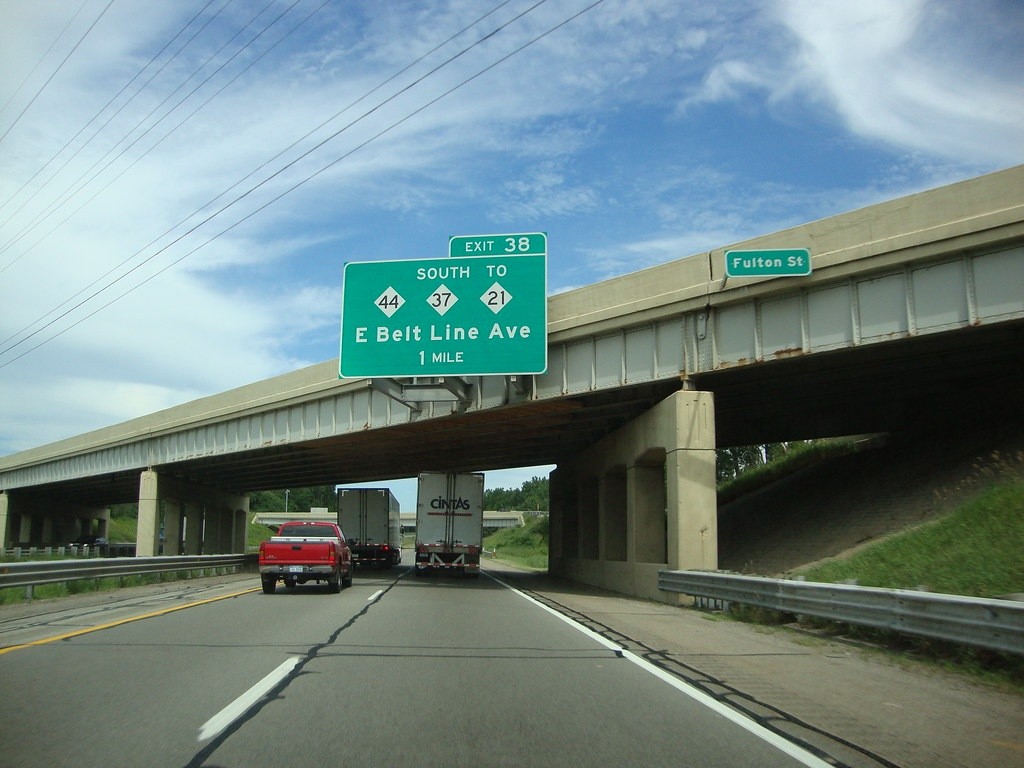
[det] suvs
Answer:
[68,536,108,552]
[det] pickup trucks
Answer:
[259,521,357,593]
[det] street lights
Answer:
[285,490,290,513]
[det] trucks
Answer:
[415,470,485,579]
[338,488,404,570]
